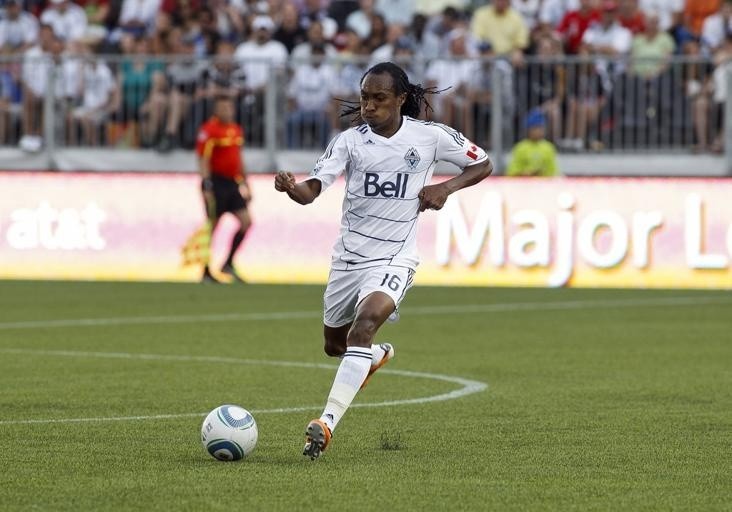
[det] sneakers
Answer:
[201,272,218,283]
[359,343,394,390]
[305,419,331,461]
[222,264,244,283]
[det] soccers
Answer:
[201,405,257,460]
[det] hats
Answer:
[252,15,277,33]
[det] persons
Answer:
[275,63,493,461]
[195,95,251,284]
